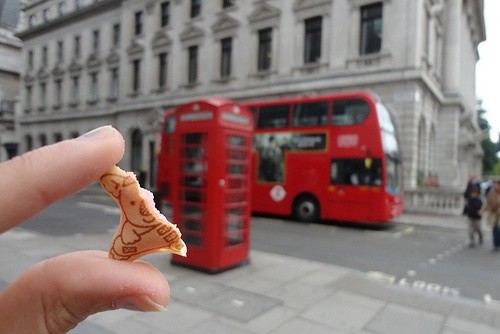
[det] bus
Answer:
[154,90,404,225]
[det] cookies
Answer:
[99,165,189,259]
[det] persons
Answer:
[463,170,500,250]
[0,125,170,333]
